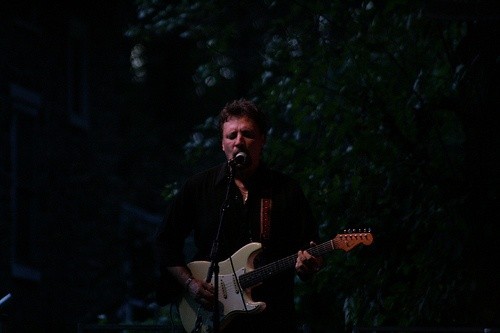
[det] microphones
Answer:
[229,151,247,167]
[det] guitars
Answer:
[178,228,373,333]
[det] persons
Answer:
[151,98,324,333]
